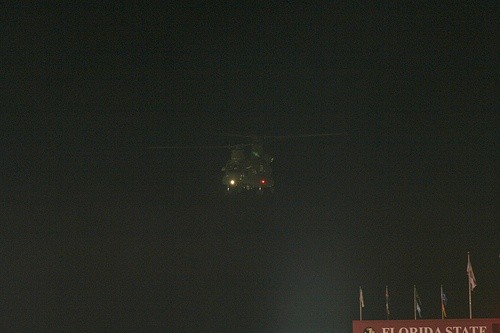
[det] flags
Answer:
[414,285,422,318]
[385,285,392,315]
[467,255,477,292]
[441,283,448,319]
[360,287,365,309]
